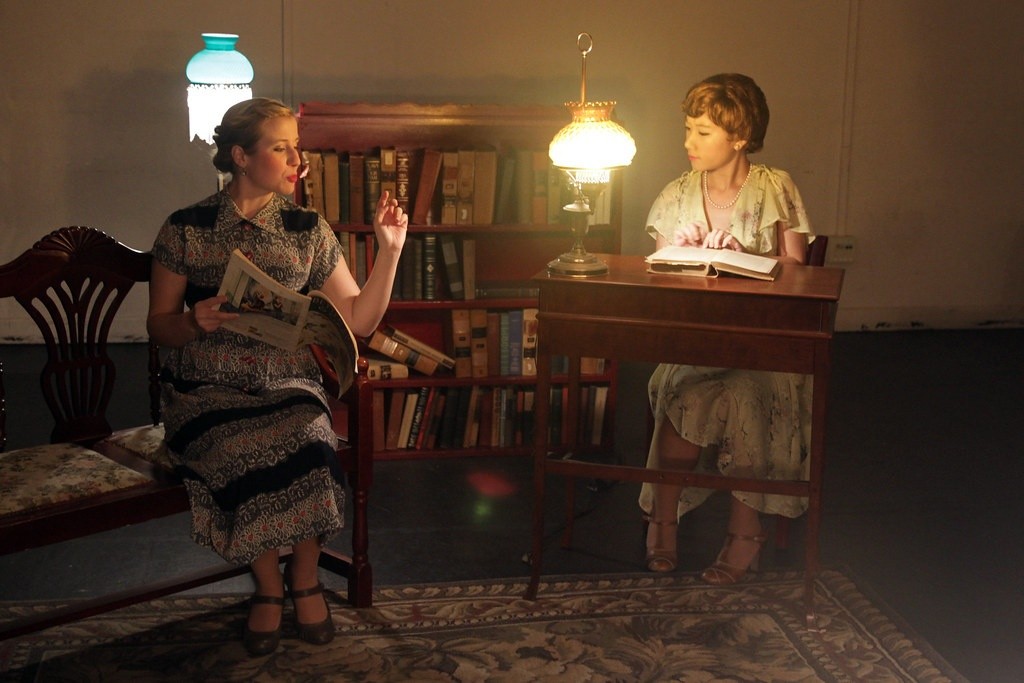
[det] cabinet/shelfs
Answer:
[292,102,623,462]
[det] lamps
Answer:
[185,33,255,191]
[548,33,637,278]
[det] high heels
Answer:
[702,521,768,586]
[643,512,678,573]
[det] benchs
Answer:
[0,227,375,643]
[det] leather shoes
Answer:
[284,553,336,646]
[244,574,285,658]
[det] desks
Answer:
[522,247,846,635]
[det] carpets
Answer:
[2,555,971,683]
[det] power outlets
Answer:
[828,235,856,262]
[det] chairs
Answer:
[642,236,830,550]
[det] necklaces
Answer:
[704,161,753,209]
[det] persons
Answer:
[633,72,817,584]
[145,97,409,656]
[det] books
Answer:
[642,244,783,282]
[295,146,615,450]
[209,248,360,404]
